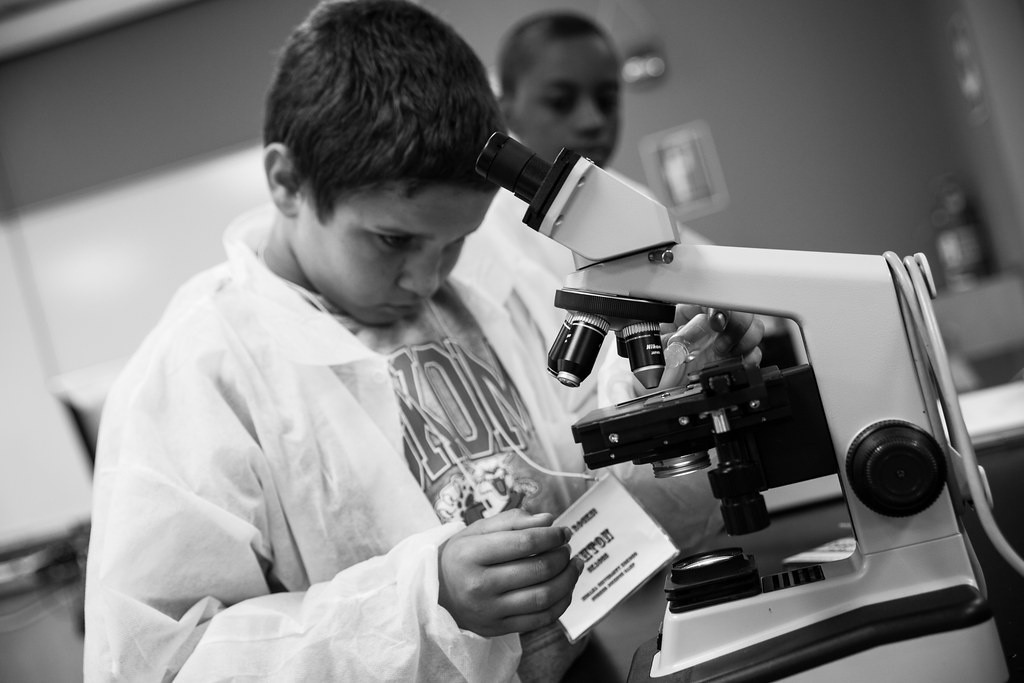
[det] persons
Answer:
[449,11,727,551]
[82,0,765,683]
